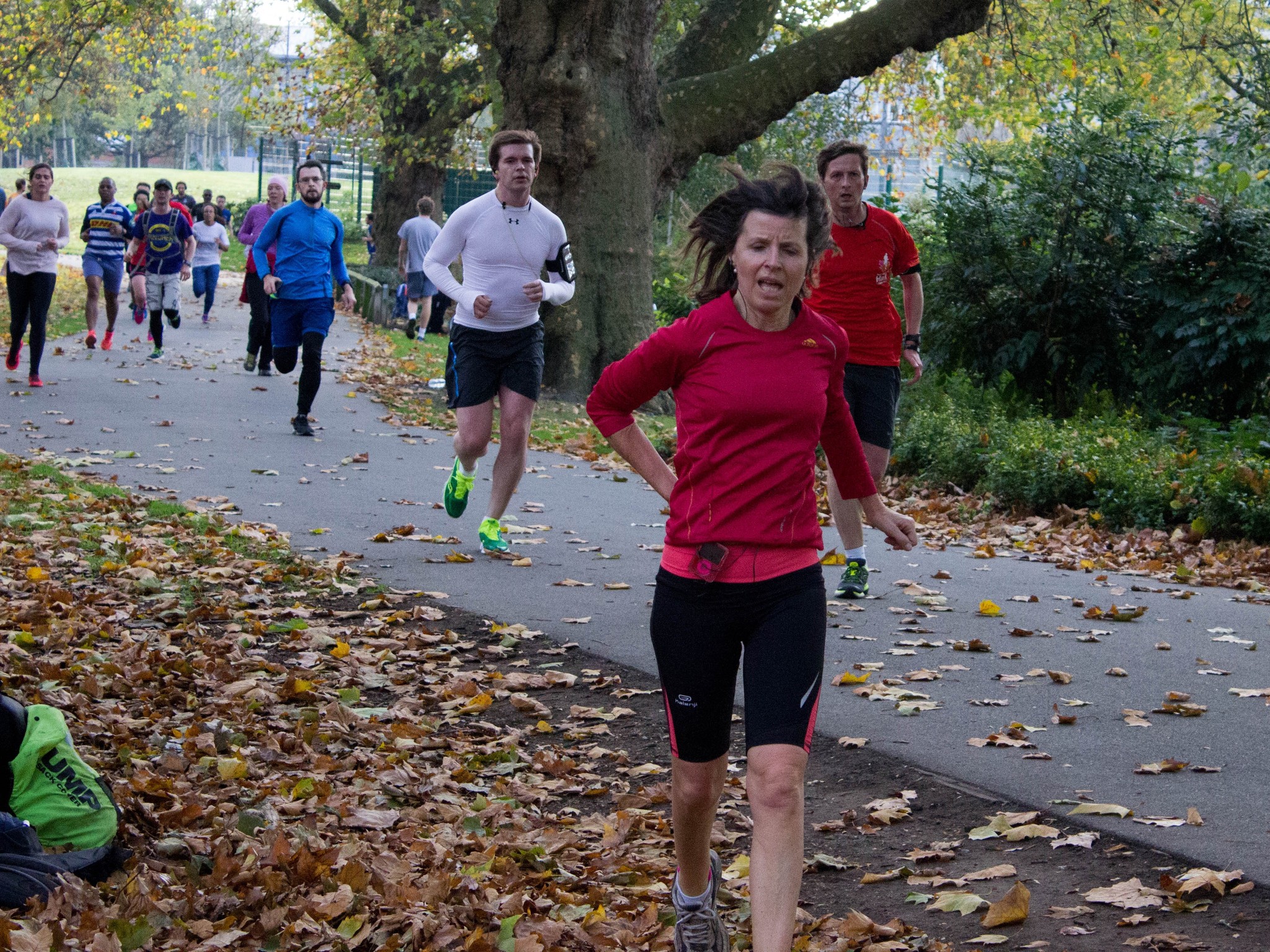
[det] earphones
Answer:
[535,165,538,173]
[492,168,498,174]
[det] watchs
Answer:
[905,333,922,345]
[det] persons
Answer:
[585,158,918,952]
[422,129,577,554]
[802,141,923,598]
[0,159,453,436]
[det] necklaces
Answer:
[832,212,863,227]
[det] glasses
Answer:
[298,177,322,184]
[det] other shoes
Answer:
[202,314,208,324]
[406,317,416,339]
[417,336,425,341]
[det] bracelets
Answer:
[341,280,353,291]
[184,260,192,267]
[904,344,920,352]
[121,226,124,234]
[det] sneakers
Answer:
[101,328,114,350]
[443,454,478,518]
[134,300,147,324]
[260,369,271,377]
[28,373,43,386]
[171,315,181,329]
[6,339,23,370]
[292,415,314,437]
[671,849,727,952]
[147,347,165,361]
[478,518,510,556]
[148,329,154,343]
[834,557,869,599]
[244,351,257,372]
[86,331,97,349]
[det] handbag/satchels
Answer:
[10,705,119,852]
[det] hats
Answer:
[155,179,172,190]
[269,177,288,198]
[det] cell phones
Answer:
[275,280,283,291]
